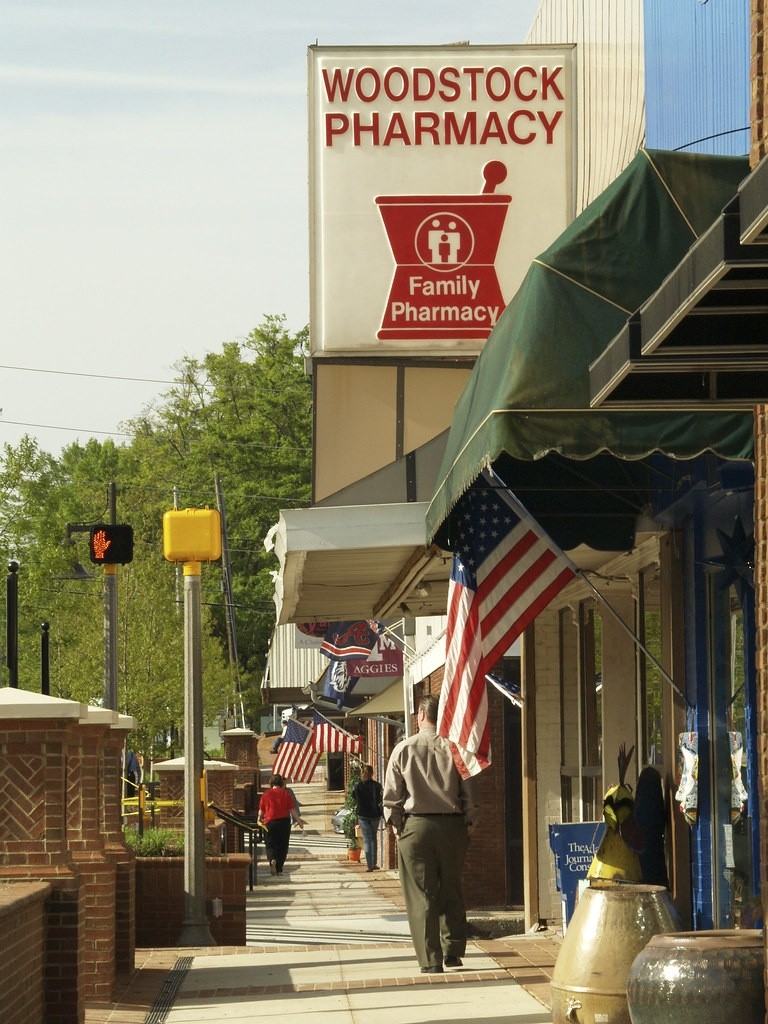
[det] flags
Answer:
[314,711,363,754]
[324,661,359,708]
[321,617,403,676]
[272,720,321,783]
[435,477,575,780]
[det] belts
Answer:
[405,812,464,817]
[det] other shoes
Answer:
[277,871,283,875]
[366,869,373,872]
[372,865,380,869]
[444,957,463,967]
[270,750,278,754]
[270,859,277,875]
[422,966,443,973]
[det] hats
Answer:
[282,720,287,724]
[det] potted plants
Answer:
[342,813,362,861]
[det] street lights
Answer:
[48,521,121,711]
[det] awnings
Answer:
[269,500,450,623]
[426,146,756,549]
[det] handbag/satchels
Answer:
[127,771,132,785]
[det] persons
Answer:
[269,720,288,754]
[257,774,303,876]
[381,693,481,974]
[352,765,384,872]
[121,746,141,797]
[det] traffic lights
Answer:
[89,524,136,566]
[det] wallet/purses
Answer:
[258,820,268,832]
